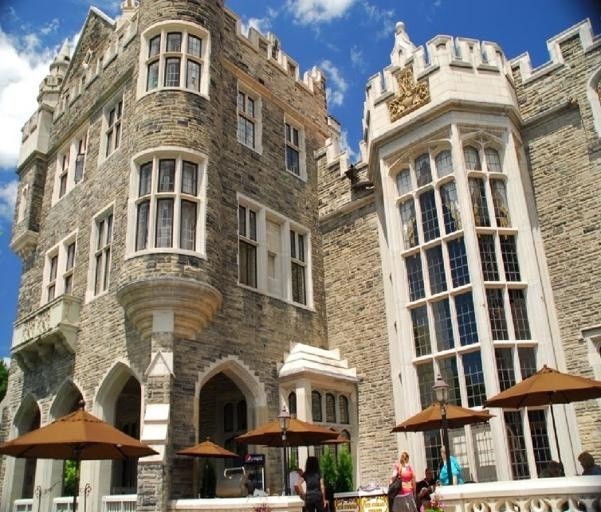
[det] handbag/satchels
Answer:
[386,464,401,497]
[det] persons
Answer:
[577,451,601,474]
[438,444,465,486]
[416,467,437,500]
[538,460,564,477]
[293,456,327,512]
[390,451,420,511]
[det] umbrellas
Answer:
[261,423,352,456]
[388,404,495,443]
[176,433,245,500]
[484,363,601,476]
[234,414,340,495]
[0,399,158,511]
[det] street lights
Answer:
[278,404,293,493]
[433,373,455,484]
